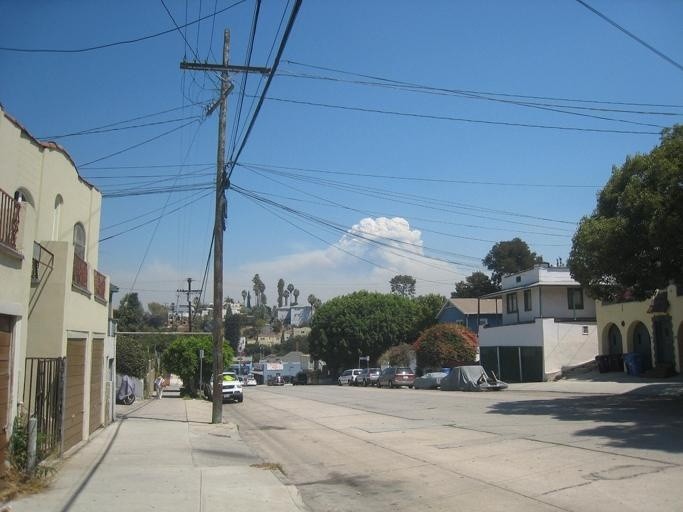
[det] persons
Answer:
[153,372,165,401]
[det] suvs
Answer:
[209,371,257,404]
[267,376,285,386]
[338,366,415,389]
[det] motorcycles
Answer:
[117,374,137,405]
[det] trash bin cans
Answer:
[595,353,622,373]
[624,353,645,376]
[276,377,282,383]
[441,367,452,375]
